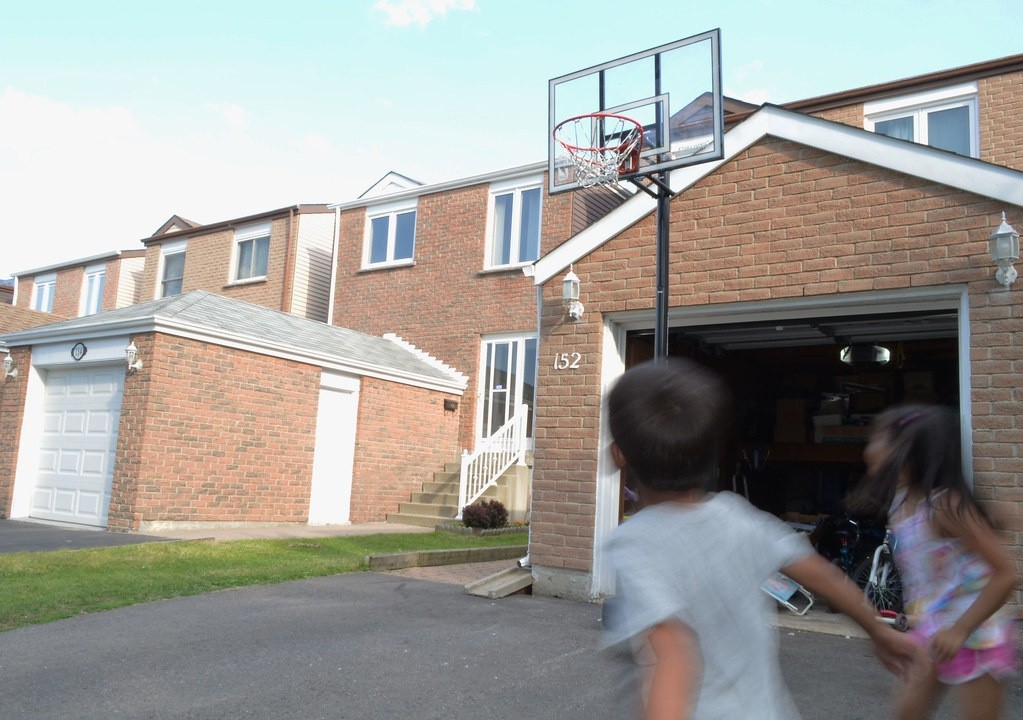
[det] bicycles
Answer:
[825,516,909,631]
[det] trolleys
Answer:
[732,469,816,617]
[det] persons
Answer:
[868,407,1023,720]
[603,364,932,720]
